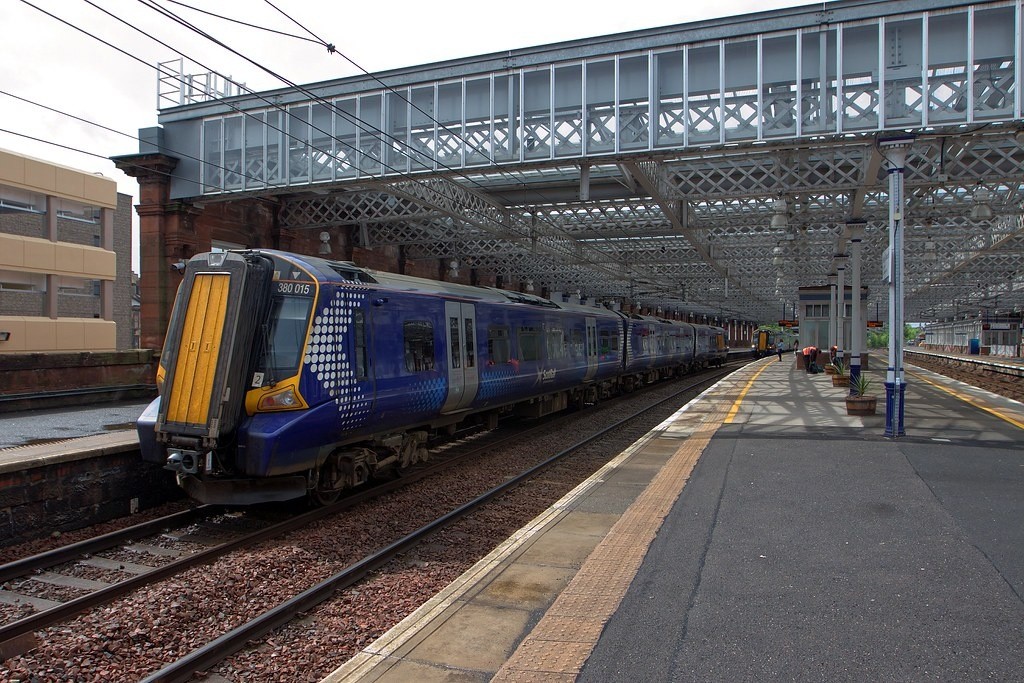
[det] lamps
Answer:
[318,232,333,254]
[448,261,458,277]
[771,191,788,228]
[972,179,992,218]
[924,235,936,250]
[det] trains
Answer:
[750,327,800,359]
[135,247,731,514]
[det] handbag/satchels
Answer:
[776,348,778,352]
[809,362,819,373]
[794,351,795,354]
[818,365,824,372]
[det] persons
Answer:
[776,338,784,362]
[793,339,799,357]
[802,346,822,372]
[829,347,841,371]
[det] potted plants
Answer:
[825,363,839,374]
[829,362,850,387]
[845,373,877,416]
